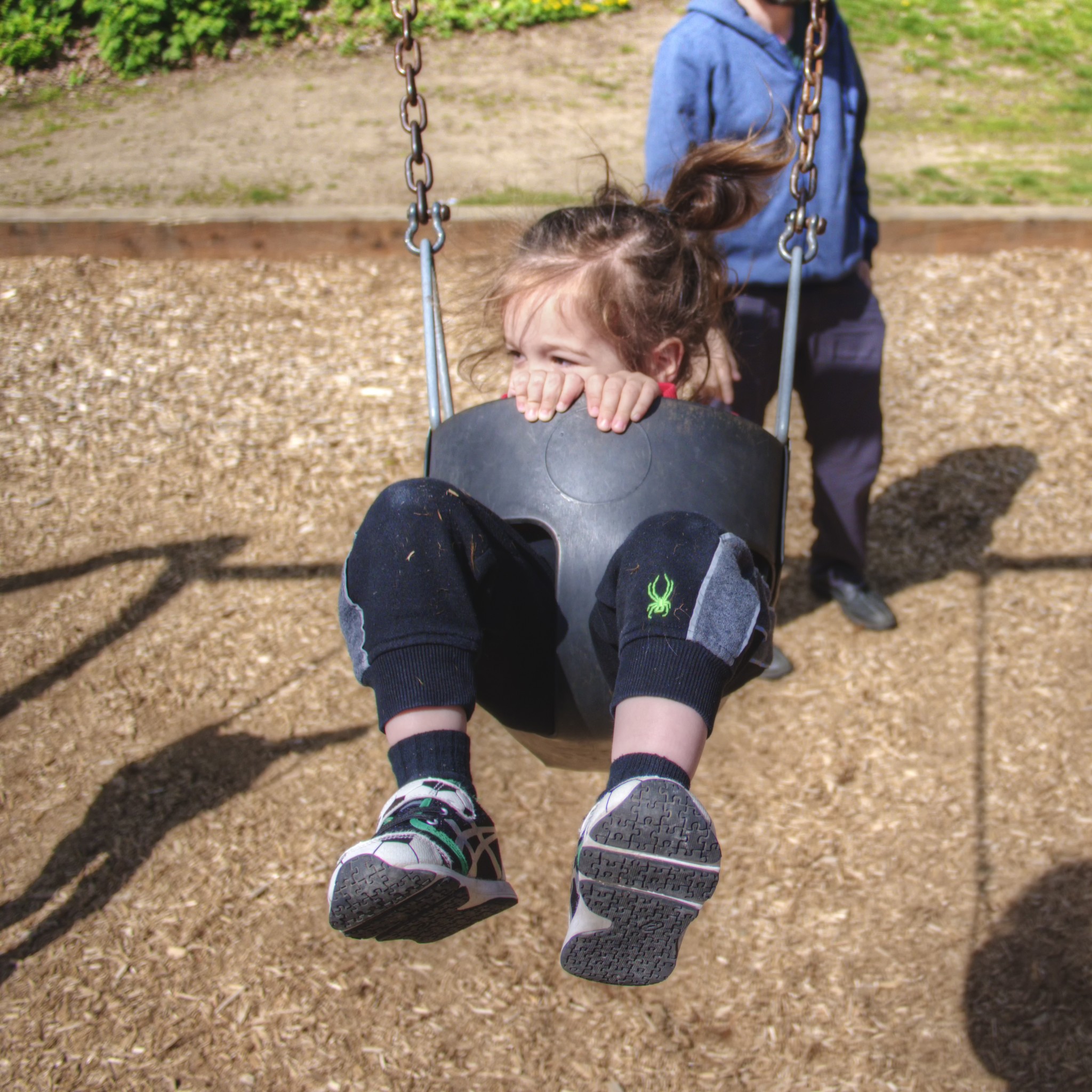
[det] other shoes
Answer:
[738,640,795,678]
[814,565,898,629]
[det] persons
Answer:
[327,127,798,987]
[646,0,899,682]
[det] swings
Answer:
[390,0,830,749]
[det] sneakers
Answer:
[561,775,722,985]
[318,802,518,944]
